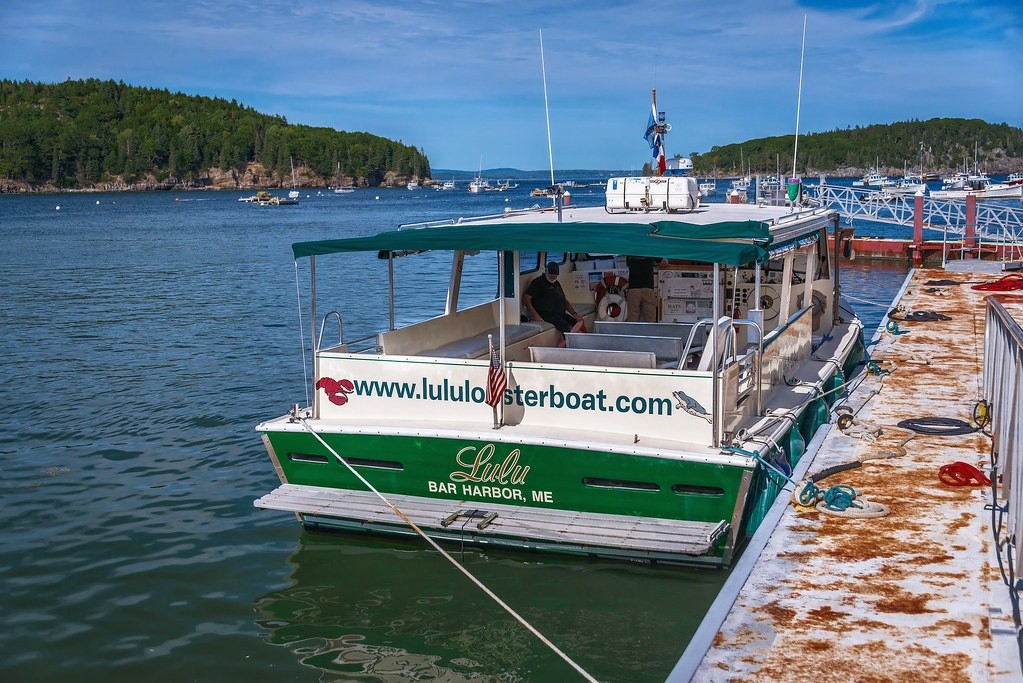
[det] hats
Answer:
[547,262,559,275]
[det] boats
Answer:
[238,13,865,570]
[852,141,1023,201]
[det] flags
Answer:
[643,104,666,175]
[485,340,507,408]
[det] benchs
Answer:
[593,320,707,357]
[378,298,540,359]
[563,332,688,370]
[520,272,597,333]
[528,346,657,369]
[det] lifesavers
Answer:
[599,293,628,322]
[594,275,631,307]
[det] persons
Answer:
[523,261,586,349]
[625,255,668,322]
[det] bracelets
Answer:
[572,312,577,316]
